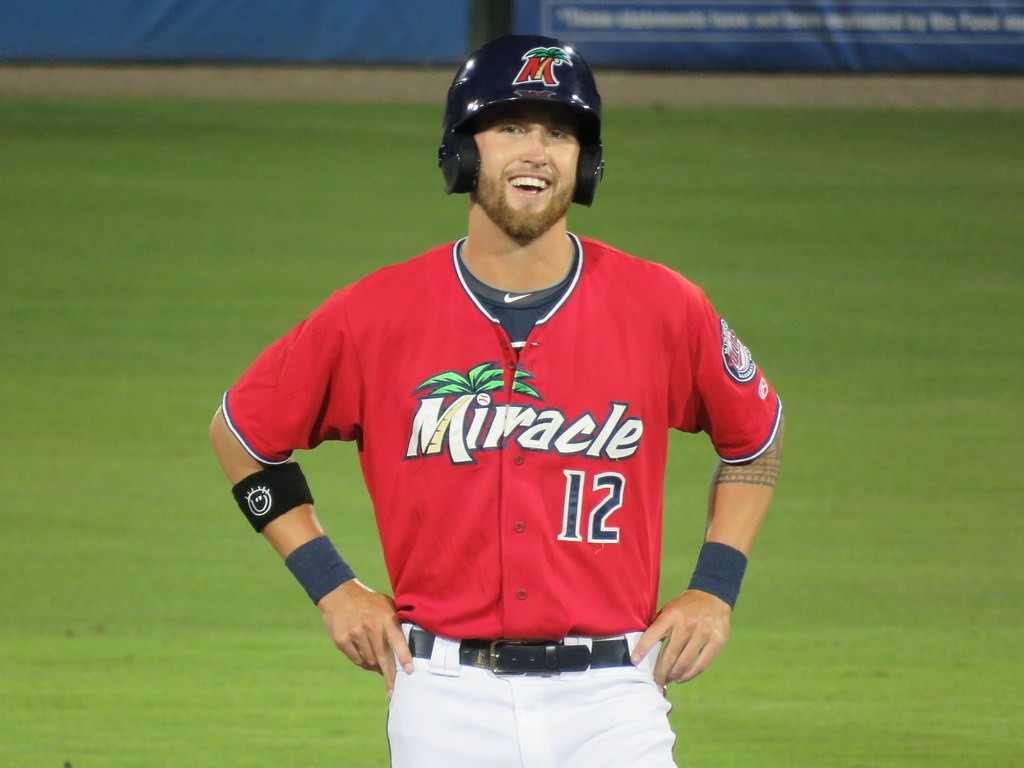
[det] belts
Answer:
[409,626,634,675]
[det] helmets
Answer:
[438,35,605,208]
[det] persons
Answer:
[209,36,783,768]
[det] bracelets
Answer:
[284,535,357,606]
[687,542,747,611]
